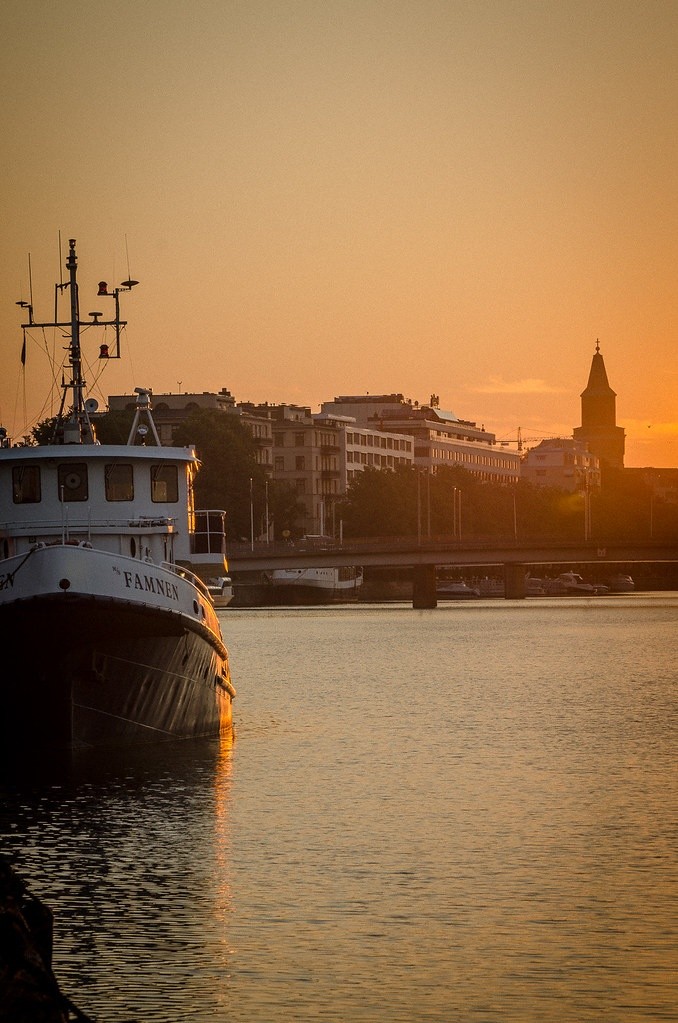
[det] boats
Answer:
[482,571,545,596]
[0,227,236,753]
[548,570,609,596]
[436,583,481,600]
[271,535,364,603]
[610,573,635,591]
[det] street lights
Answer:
[410,463,427,548]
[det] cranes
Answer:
[495,426,576,457]
